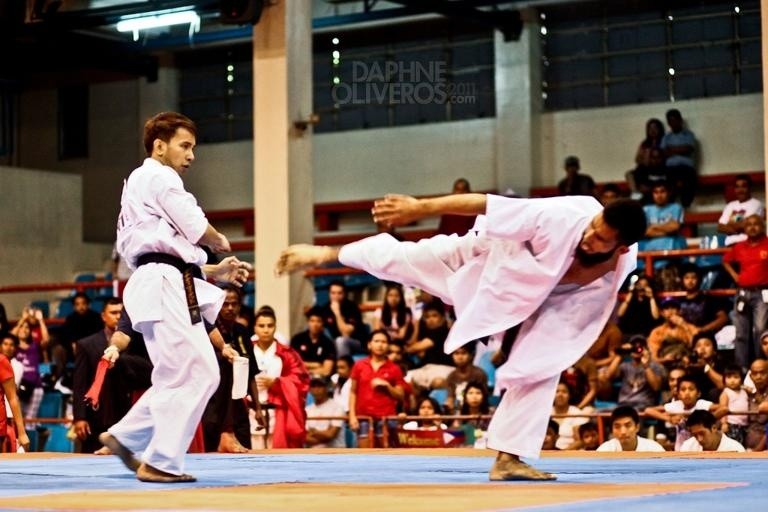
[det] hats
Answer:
[629,334,646,344]
[660,296,680,307]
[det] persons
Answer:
[274,194,650,481]
[541,109,768,453]
[1,241,500,456]
[99,111,254,482]
[438,178,477,236]
